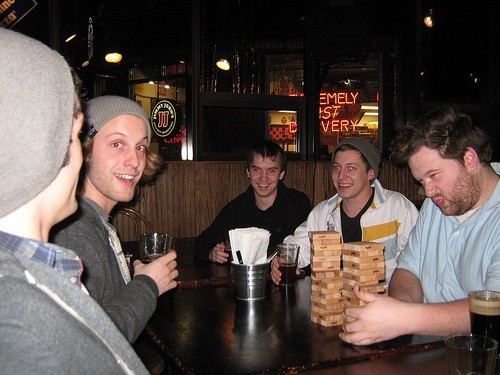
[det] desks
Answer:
[119,236,471,375]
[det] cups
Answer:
[444,332,499,375]
[230,260,268,301]
[467,290,500,375]
[138,233,174,265]
[275,244,302,286]
[222,239,233,262]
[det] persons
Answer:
[0,27,150,375]
[271,138,419,285]
[194,139,311,264]
[339,104,500,346]
[48,95,179,346]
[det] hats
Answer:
[0,28,73,219]
[332,137,380,185]
[80,95,151,149]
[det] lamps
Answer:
[215,56,233,71]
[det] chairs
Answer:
[111,206,155,240]
